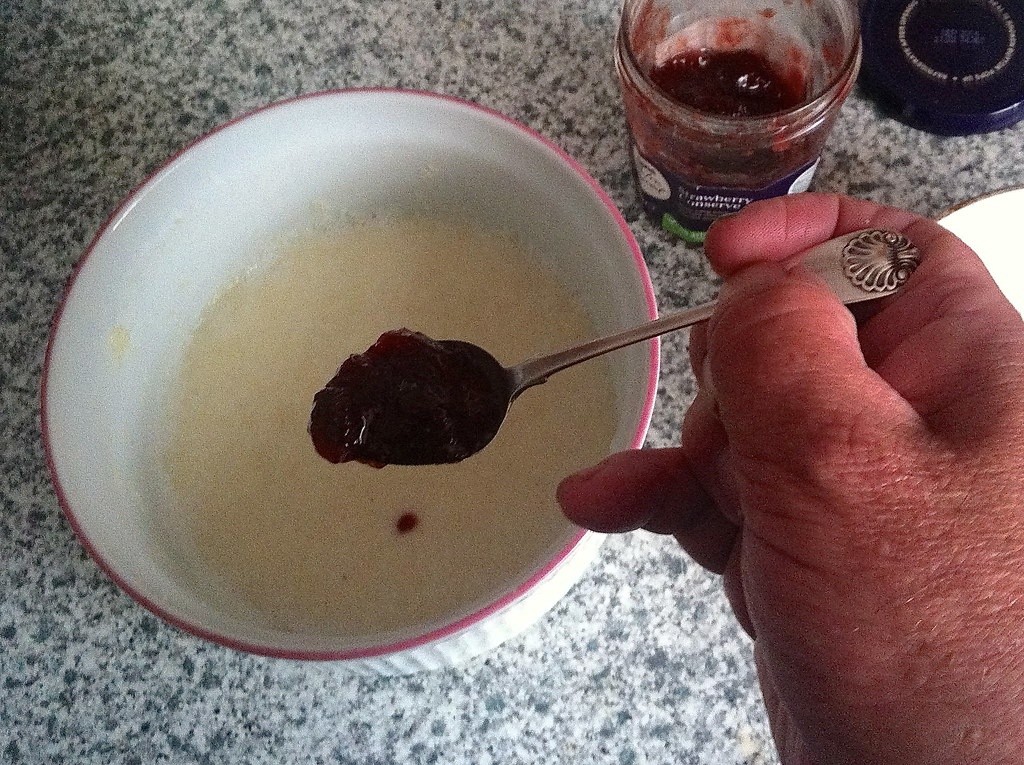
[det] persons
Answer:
[553,193,1024,765]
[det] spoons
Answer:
[380,228,918,466]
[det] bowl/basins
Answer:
[42,85,661,671]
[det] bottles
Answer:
[614,0,863,244]
